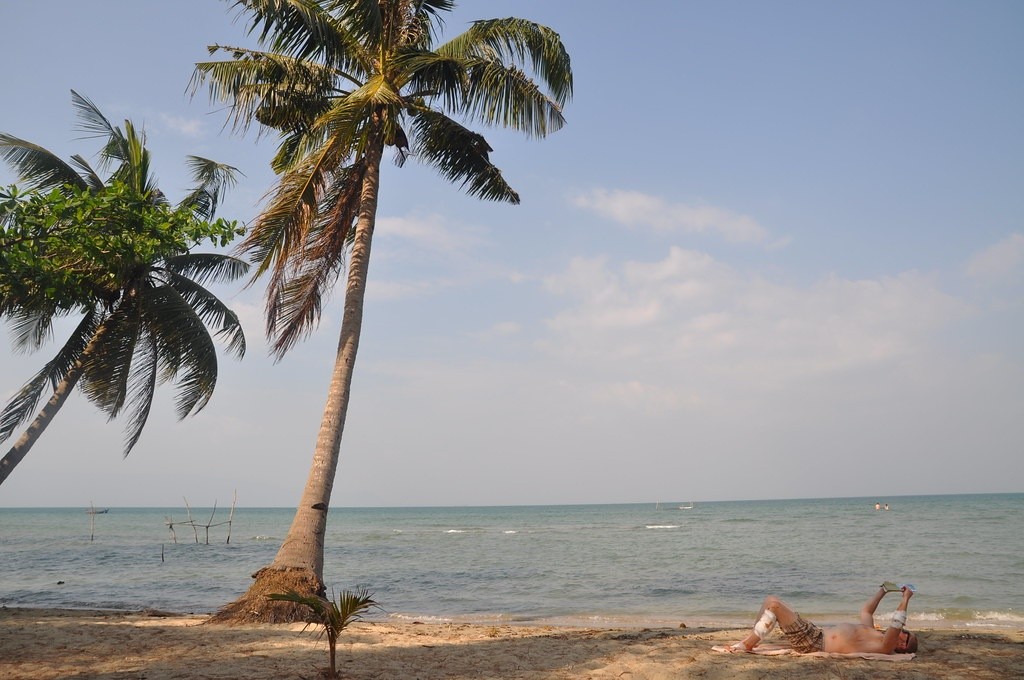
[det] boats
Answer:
[678,506,692,510]
[87,507,109,514]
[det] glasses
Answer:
[903,630,910,646]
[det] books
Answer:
[881,581,916,592]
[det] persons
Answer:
[875,502,880,509]
[724,586,918,654]
[885,504,889,510]
